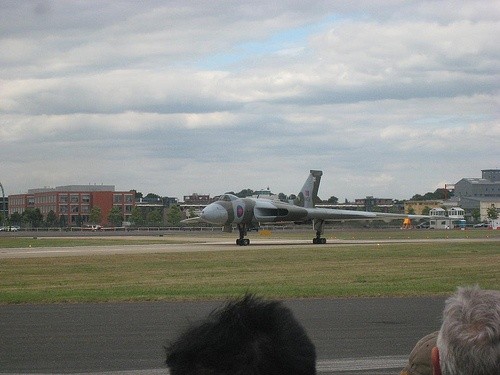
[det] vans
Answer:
[5,226,17,232]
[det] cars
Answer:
[417,221,430,228]
[180,169,466,245]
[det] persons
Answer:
[402,331,441,375]
[165,289,317,375]
[430,284,500,375]
